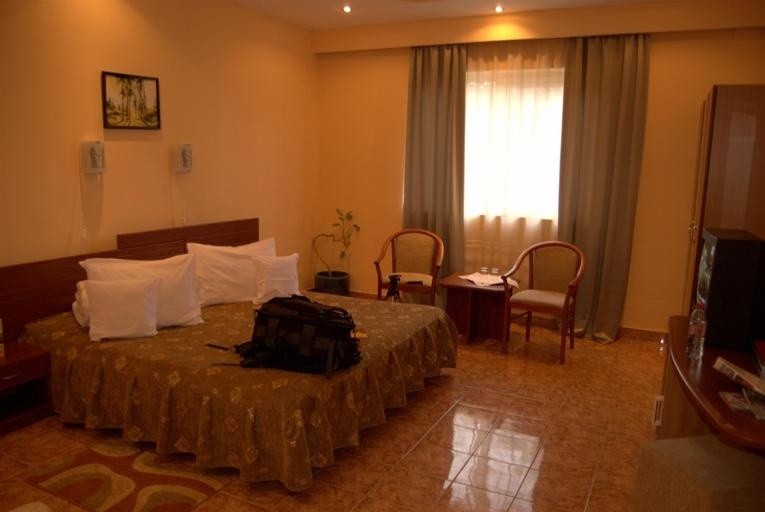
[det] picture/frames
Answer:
[102,71,160,129]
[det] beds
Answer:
[25,277,453,485]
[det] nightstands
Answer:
[0,342,53,438]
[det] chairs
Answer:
[374,227,444,307]
[500,240,588,365]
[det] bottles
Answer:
[686,303,707,361]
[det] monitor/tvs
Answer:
[689,227,765,352]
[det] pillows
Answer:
[73,234,303,345]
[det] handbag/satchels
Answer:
[236,294,363,376]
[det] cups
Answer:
[478,266,500,286]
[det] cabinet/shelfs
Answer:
[652,335,722,438]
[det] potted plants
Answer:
[311,206,361,296]
